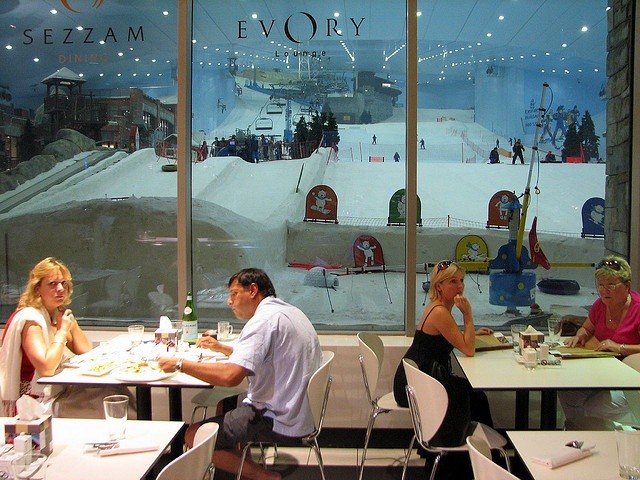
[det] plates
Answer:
[109,359,179,382]
[80,350,128,376]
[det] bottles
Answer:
[182,290,198,345]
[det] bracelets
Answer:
[618,344,629,357]
[50,339,67,347]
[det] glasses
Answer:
[437,260,453,274]
[597,282,624,292]
[596,260,620,271]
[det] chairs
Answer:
[466,436,520,480]
[190,386,248,424]
[237,351,334,480]
[157,422,220,480]
[356,333,410,480]
[402,358,510,479]
[567,144,585,163]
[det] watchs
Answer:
[174,358,184,373]
[158,266,322,480]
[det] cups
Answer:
[10,452,49,480]
[218,320,233,339]
[128,325,144,347]
[614,424,640,480]
[102,394,130,441]
[547,318,563,346]
[511,324,527,354]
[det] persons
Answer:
[550,105,567,149]
[419,138,426,150]
[539,114,557,142]
[370,134,378,145]
[508,138,513,146]
[391,258,495,480]
[544,148,556,161]
[200,139,210,160]
[211,133,283,164]
[0,257,135,426]
[561,108,577,141]
[557,254,640,431]
[510,138,526,164]
[392,151,401,163]
[488,146,501,163]
[570,105,582,132]
[512,136,518,145]
[496,138,500,148]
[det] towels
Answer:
[96,438,158,456]
[531,441,595,469]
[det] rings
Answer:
[602,342,607,346]
